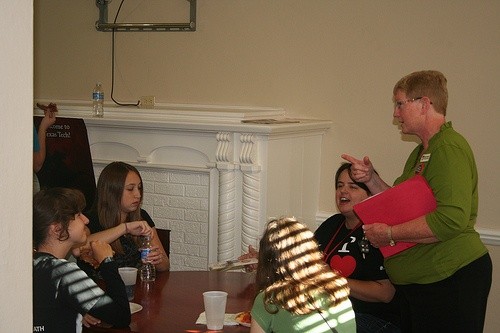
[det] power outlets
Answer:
[135,95,156,109]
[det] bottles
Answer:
[92,84,104,118]
[140,236,156,282]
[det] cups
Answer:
[118,267,137,301]
[202,291,228,330]
[140,96,156,109]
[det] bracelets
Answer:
[124,222,128,236]
[99,257,114,266]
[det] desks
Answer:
[129,270,266,333]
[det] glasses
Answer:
[395,96,433,109]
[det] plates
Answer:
[129,302,143,314]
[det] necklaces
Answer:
[322,218,362,262]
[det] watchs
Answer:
[387,225,397,247]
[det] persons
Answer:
[249,217,356,333]
[33,109,55,194]
[238,163,395,333]
[33,188,131,333]
[341,70,493,333]
[72,162,170,283]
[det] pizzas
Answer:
[235,311,251,323]
